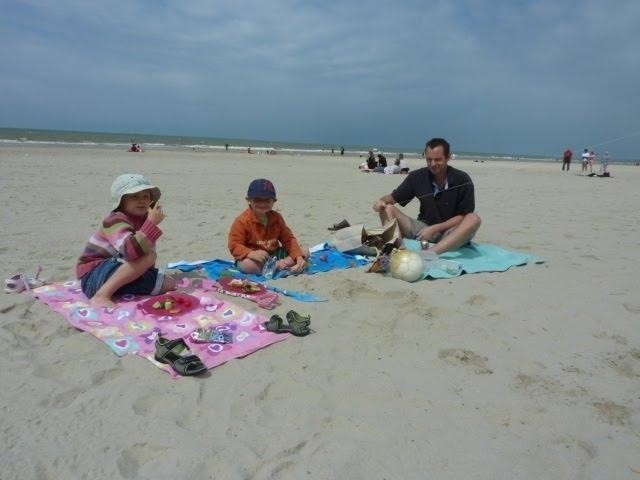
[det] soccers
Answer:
[391,249,423,282]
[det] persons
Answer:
[561,148,572,172]
[601,151,609,173]
[341,146,344,155]
[77,174,178,310]
[588,152,596,172]
[131,144,143,152]
[372,138,481,255]
[247,146,250,153]
[227,178,310,278]
[225,143,228,150]
[363,150,409,175]
[581,148,590,172]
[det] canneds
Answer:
[422,239,428,250]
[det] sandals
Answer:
[191,325,233,343]
[154,337,207,376]
[4,264,46,294]
[327,219,350,231]
[260,310,312,337]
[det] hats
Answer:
[110,173,162,212]
[245,178,278,202]
